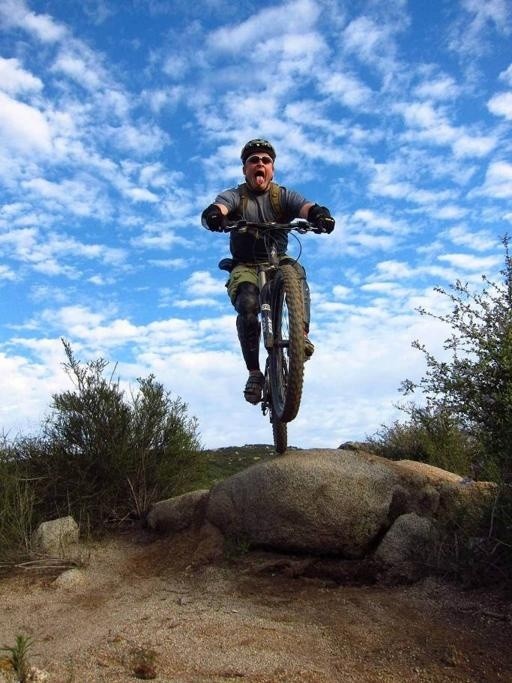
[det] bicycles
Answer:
[212,218,325,455]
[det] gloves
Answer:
[306,202,336,234]
[200,202,225,233]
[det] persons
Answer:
[201,139,334,405]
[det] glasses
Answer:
[244,154,274,165]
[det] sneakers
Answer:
[242,369,266,405]
[286,335,315,358]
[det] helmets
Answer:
[240,137,277,164]
